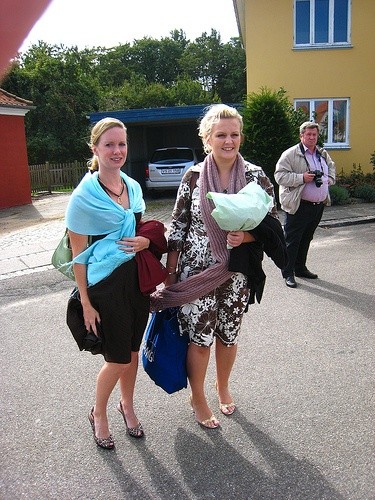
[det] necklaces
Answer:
[105,185,124,205]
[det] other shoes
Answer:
[285,275,297,287]
[295,269,318,278]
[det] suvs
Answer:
[144,146,198,196]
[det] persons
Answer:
[66,117,150,450]
[164,104,279,428]
[274,122,335,288]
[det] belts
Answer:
[301,195,328,205]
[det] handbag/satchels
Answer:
[141,311,188,394]
[52,228,92,283]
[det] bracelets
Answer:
[168,266,176,275]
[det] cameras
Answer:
[308,169,324,187]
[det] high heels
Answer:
[187,394,219,428]
[116,401,144,438]
[88,407,115,450]
[215,383,235,415]
